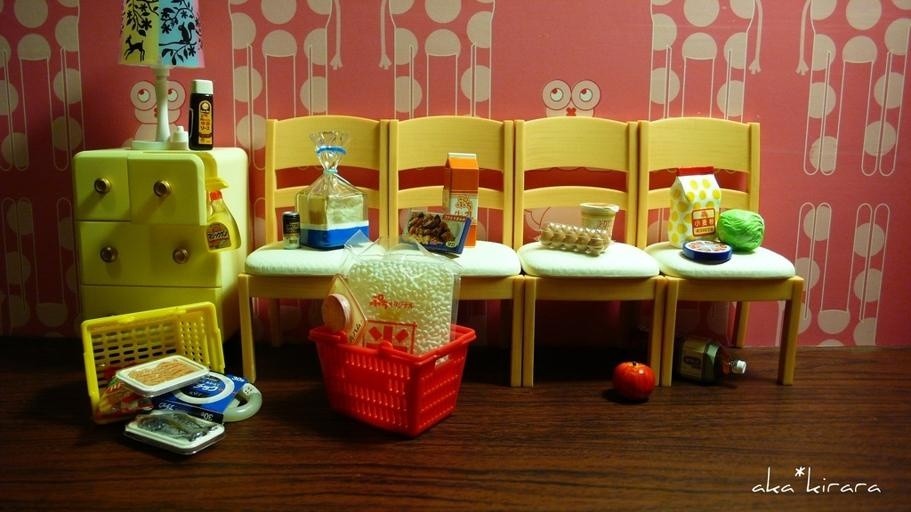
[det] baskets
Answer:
[307,316,478,438]
[80,301,227,427]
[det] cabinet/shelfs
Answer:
[74,147,249,377]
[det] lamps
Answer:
[116,0,206,149]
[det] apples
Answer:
[613,360,656,398]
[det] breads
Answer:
[296,190,364,248]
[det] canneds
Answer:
[282,211,300,249]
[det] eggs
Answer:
[541,223,609,253]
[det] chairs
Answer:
[237,115,390,384]
[638,116,804,387]
[514,116,667,388]
[390,115,525,388]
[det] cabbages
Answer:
[717,208,765,252]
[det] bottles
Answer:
[624,331,746,382]
[188,79,213,150]
